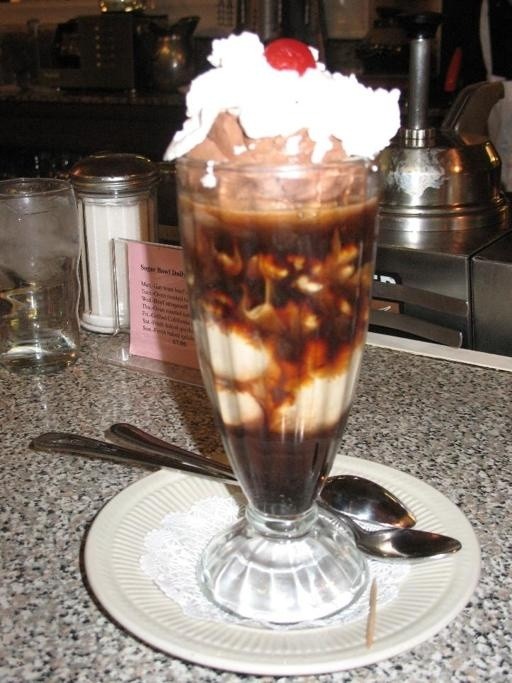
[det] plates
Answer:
[78,449,483,674]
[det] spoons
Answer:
[29,430,462,562]
[109,423,417,530]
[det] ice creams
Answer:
[164,30,402,526]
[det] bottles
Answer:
[70,152,156,332]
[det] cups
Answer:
[175,147,377,629]
[0,175,83,378]
